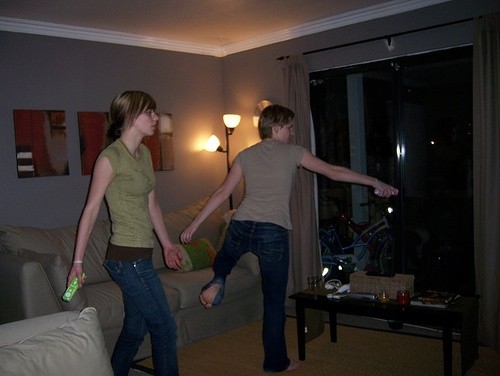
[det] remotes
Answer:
[375,185,399,198]
[62,272,87,301]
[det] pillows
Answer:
[172,236,218,272]
[21,247,91,310]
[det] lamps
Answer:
[203,114,241,211]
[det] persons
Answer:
[66,90,184,375]
[179,104,399,373]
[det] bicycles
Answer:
[314,192,400,280]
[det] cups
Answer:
[308,276,319,289]
[378,290,389,307]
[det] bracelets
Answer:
[72,261,83,264]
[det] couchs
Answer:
[0,195,263,362]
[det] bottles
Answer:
[395,290,411,314]
